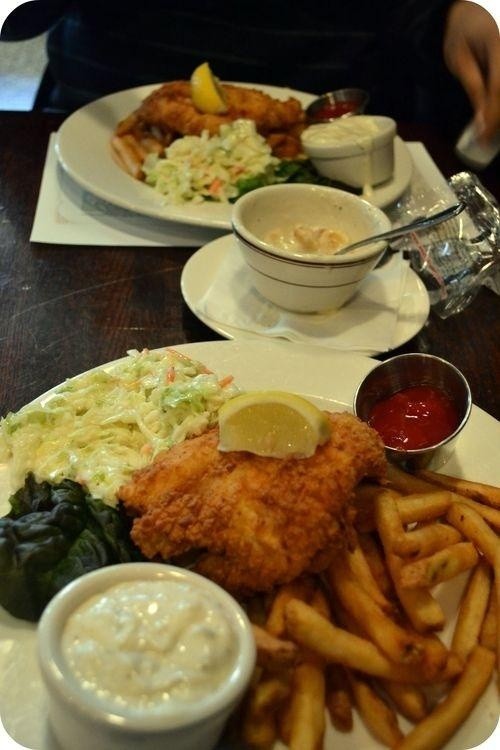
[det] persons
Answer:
[0,2,499,143]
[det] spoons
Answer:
[326,204,468,260]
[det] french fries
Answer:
[245,464,500,750]
[112,129,171,179]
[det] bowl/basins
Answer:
[356,354,470,456]
[301,87,375,131]
[299,116,398,189]
[32,558,258,750]
[234,184,387,315]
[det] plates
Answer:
[55,80,416,230]
[0,340,500,746]
[180,230,432,359]
